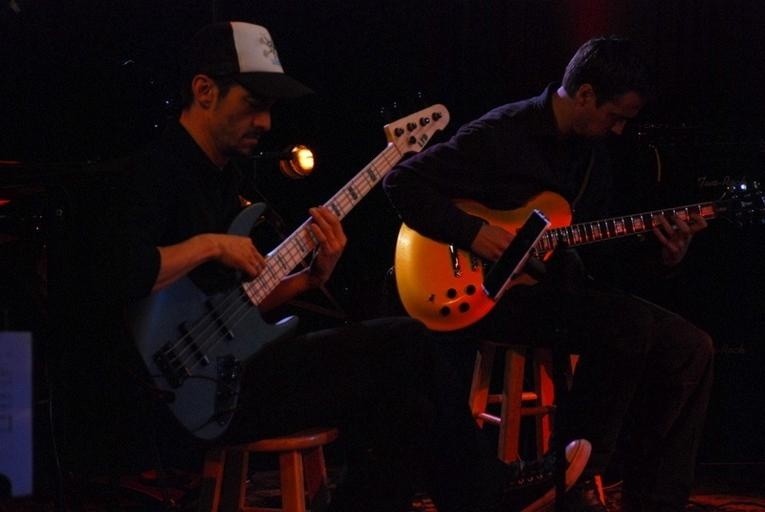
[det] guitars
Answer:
[395,181,764,332]
[132,104,450,440]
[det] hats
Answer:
[185,19,315,105]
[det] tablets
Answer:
[481,209,552,302]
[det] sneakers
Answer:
[473,438,593,510]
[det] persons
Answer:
[382,36,713,512]
[62,21,593,512]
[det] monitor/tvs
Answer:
[0,323,37,502]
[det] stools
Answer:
[466,330,557,463]
[209,425,343,511]
